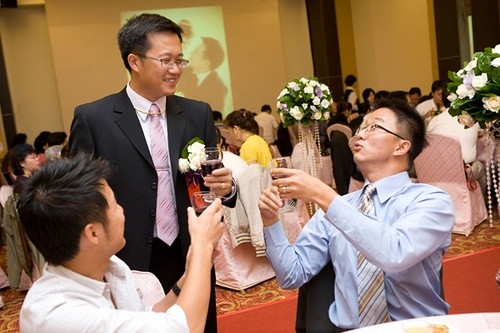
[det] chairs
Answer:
[211,124,361,295]
[412,134,489,237]
[0,182,40,294]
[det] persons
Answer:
[415,79,448,124]
[426,87,485,185]
[254,105,278,145]
[328,74,392,129]
[408,87,421,104]
[223,110,272,168]
[176,36,228,110]
[213,110,240,155]
[9,144,40,197]
[8,133,26,181]
[61,13,238,333]
[258,101,454,333]
[34,131,67,156]
[17,151,223,333]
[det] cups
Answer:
[192,191,216,218]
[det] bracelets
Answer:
[172,283,181,297]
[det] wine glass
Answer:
[268,158,298,214]
[200,147,223,203]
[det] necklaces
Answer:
[134,107,165,114]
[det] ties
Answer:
[356,185,391,327]
[147,104,180,247]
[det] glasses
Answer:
[26,155,39,160]
[132,53,189,70]
[355,123,405,146]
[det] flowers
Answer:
[443,43,500,129]
[274,76,334,128]
[178,137,209,174]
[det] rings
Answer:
[283,187,286,193]
[222,183,225,188]
